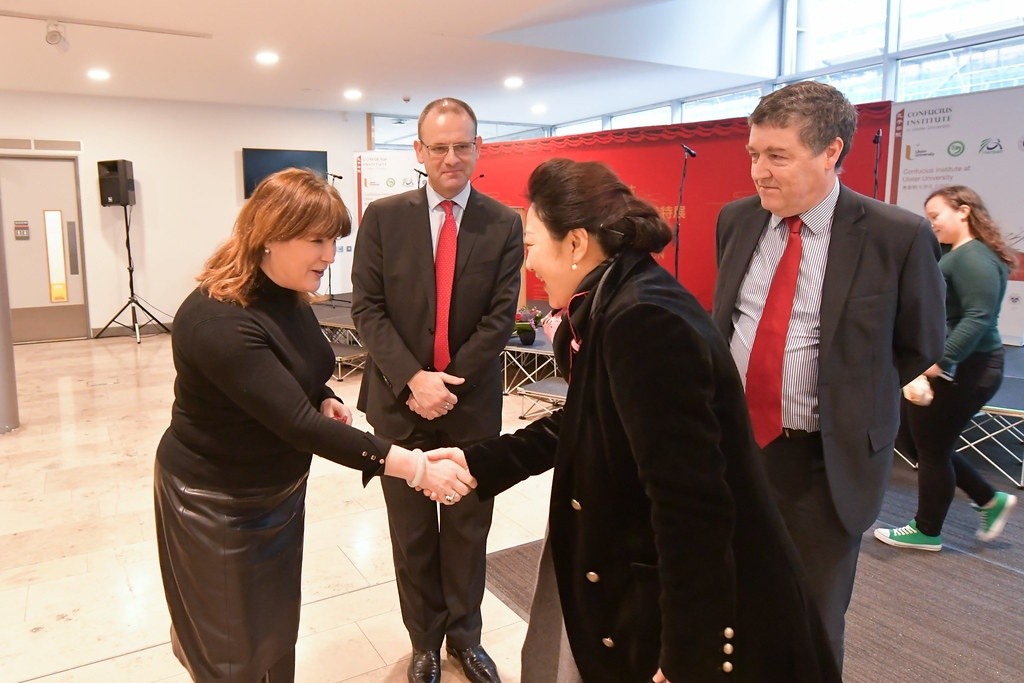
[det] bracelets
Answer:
[406,448,425,488]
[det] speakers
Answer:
[97,159,137,207]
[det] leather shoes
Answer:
[447,641,502,683]
[407,638,441,683]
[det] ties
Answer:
[745,214,804,448]
[433,200,458,373]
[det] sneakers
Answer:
[969,492,1018,542]
[875,517,943,552]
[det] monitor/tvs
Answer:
[243,148,329,200]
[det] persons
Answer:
[712,80,947,683]
[350,98,524,683]
[154,167,478,683]
[874,185,1017,551]
[416,159,842,683]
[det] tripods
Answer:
[94,206,170,344]
[322,177,351,309]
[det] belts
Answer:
[781,425,821,441]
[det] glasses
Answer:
[418,137,476,156]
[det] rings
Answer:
[445,491,456,502]
[444,402,449,409]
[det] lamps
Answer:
[45,19,63,44]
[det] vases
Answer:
[518,327,535,345]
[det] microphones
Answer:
[471,174,484,183]
[874,128,883,144]
[680,143,697,158]
[326,172,343,179]
[414,168,427,177]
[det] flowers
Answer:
[516,306,542,327]
